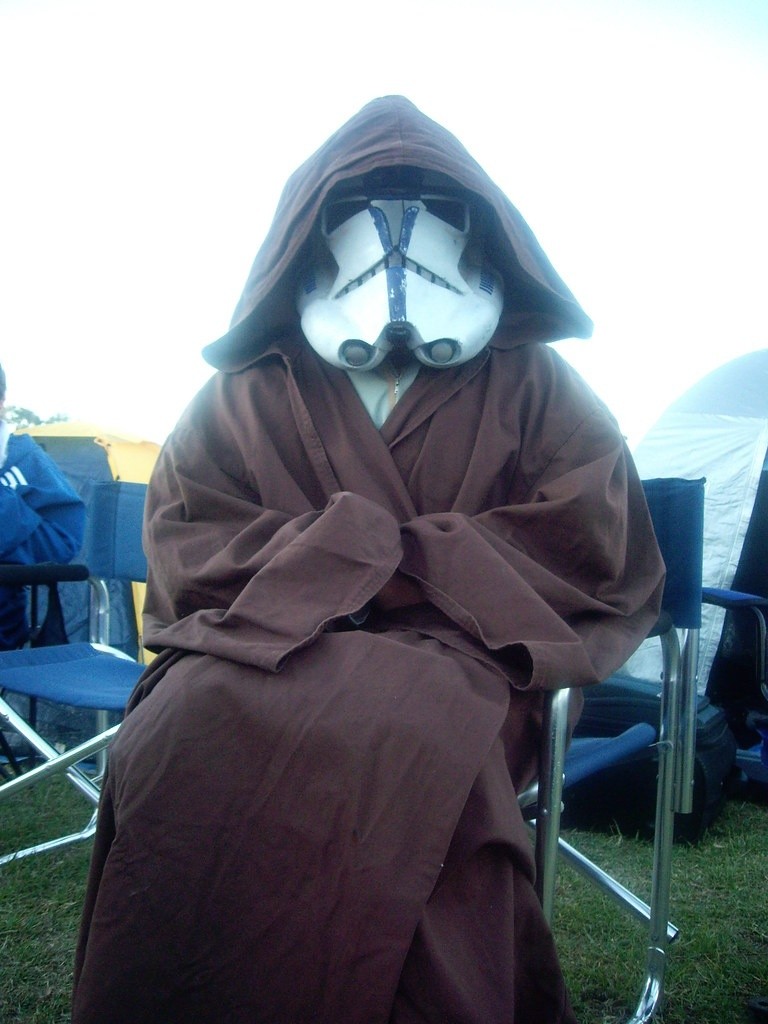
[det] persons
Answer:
[0,365,86,650]
[75,96,668,1024]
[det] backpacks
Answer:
[564,673,737,843]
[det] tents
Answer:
[613,348,768,697]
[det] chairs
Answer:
[0,480,150,868]
[516,410,768,1024]
